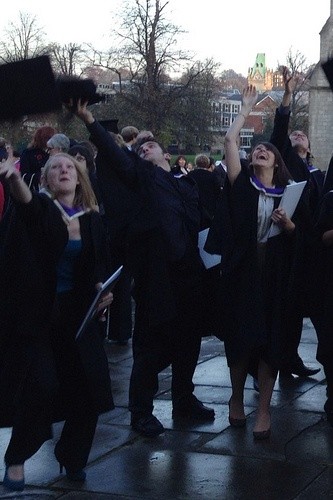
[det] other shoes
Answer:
[252,414,272,439]
[130,412,164,435]
[172,400,215,418]
[106,337,128,346]
[228,397,247,427]
[290,365,322,377]
[278,372,291,388]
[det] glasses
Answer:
[46,147,52,154]
[73,156,85,162]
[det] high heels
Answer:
[57,457,85,481]
[3,465,26,491]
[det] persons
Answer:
[0,152,115,491]
[68,97,215,435]
[0,126,251,345]
[221,84,333,440]
